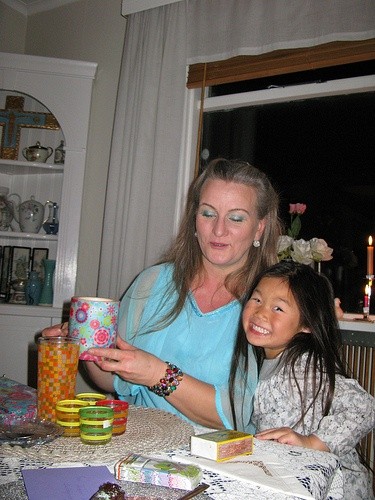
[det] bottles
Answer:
[25,270,40,306]
[42,202,59,235]
[53,141,65,163]
[37,259,56,306]
[0,186,13,231]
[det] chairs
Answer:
[332,330,375,476]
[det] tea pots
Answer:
[11,195,49,233]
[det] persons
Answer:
[43,160,282,432]
[228,259,375,500]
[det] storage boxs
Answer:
[190,429,253,462]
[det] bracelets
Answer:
[149,361,183,397]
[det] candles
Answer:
[367,235,374,275]
[364,284,369,307]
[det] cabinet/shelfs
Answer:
[0,51,99,393]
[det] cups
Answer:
[67,296,118,360]
[36,336,81,427]
[79,407,114,444]
[56,400,89,437]
[96,400,128,436]
[74,391,108,407]
[22,141,54,163]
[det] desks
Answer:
[0,389,344,500]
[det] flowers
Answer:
[271,202,334,265]
